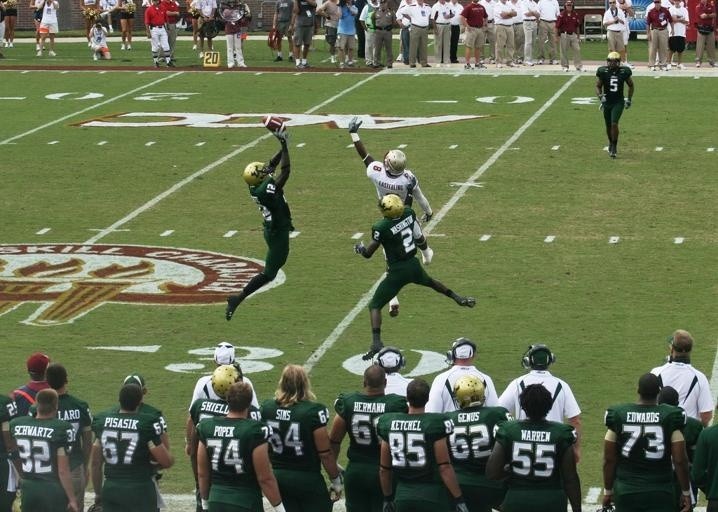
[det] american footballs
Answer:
[261,116,289,135]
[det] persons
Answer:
[603,330,718,512]
[224,128,297,322]
[646,0,717,70]
[594,51,635,159]
[79,1,136,61]
[187,339,581,512]
[0,356,173,512]
[1,1,60,57]
[603,0,634,65]
[347,115,434,318]
[143,1,251,68]
[273,0,583,71]
[352,192,477,361]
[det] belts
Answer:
[376,19,666,35]
[152,26,165,28]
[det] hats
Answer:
[530,345,550,365]
[666,335,692,352]
[26,353,49,377]
[124,372,145,389]
[214,342,235,365]
[452,337,474,359]
[379,346,401,369]
[380,0,386,3]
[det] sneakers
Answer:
[609,145,612,152]
[225,295,239,321]
[609,150,617,157]
[192,43,248,69]
[273,43,316,68]
[154,45,176,69]
[420,247,433,265]
[389,296,400,317]
[4,42,132,61]
[622,61,718,71]
[331,53,585,73]
[362,343,384,361]
[457,296,476,308]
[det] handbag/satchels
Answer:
[699,29,711,35]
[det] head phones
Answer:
[446,337,477,362]
[372,346,406,368]
[521,343,556,370]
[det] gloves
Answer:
[348,116,363,133]
[420,213,433,222]
[273,124,287,143]
[353,241,366,256]
[453,494,469,511]
[326,473,343,502]
[625,99,631,109]
[599,94,607,103]
[382,495,394,511]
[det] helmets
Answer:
[378,193,404,220]
[212,362,243,399]
[452,376,486,409]
[243,162,266,187]
[383,149,406,176]
[607,52,621,61]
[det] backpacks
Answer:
[268,29,283,50]
[195,16,219,38]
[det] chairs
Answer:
[584,14,603,43]
